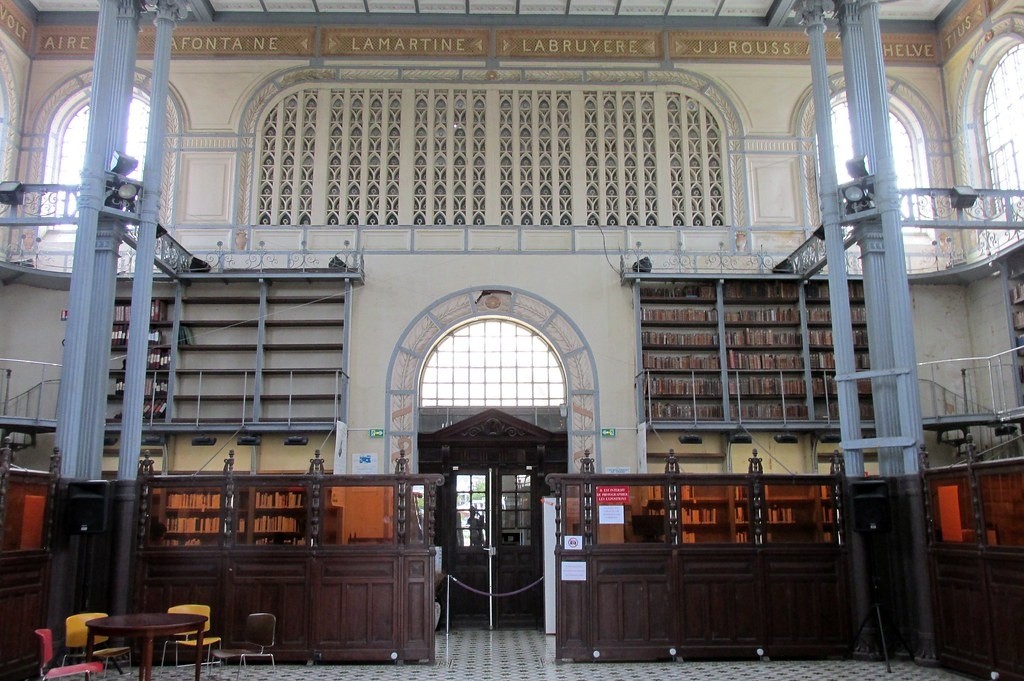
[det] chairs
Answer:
[34,629,104,681]
[207,612,277,681]
[160,604,222,680]
[58,613,133,680]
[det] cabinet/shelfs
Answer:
[647,450,878,541]
[984,545,1024,681]
[555,543,678,663]
[633,293,875,422]
[762,543,853,659]
[314,545,436,665]
[137,549,224,665]
[105,295,345,423]
[220,548,312,661]
[927,542,993,681]
[680,543,758,661]
[150,484,343,547]
[1008,269,1024,384]
[0,548,54,681]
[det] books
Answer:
[640,305,874,420]
[766,506,794,542]
[630,486,664,515]
[820,486,835,542]
[236,487,303,546]
[680,484,717,544]
[734,486,750,543]
[765,485,769,500]
[165,493,225,548]
[105,302,170,420]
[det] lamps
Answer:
[631,256,652,272]
[862,429,876,438]
[283,436,308,445]
[774,430,799,443]
[328,255,348,268]
[156,223,168,239]
[846,154,870,178]
[237,436,261,446]
[772,257,794,273]
[678,430,702,444]
[111,174,141,201]
[191,436,216,446]
[190,256,212,272]
[995,423,1017,436]
[110,151,140,176]
[104,437,118,445]
[730,430,752,444]
[0,180,25,206]
[141,436,165,445]
[949,185,978,209]
[819,429,842,443]
[842,183,869,213]
[813,225,825,240]
